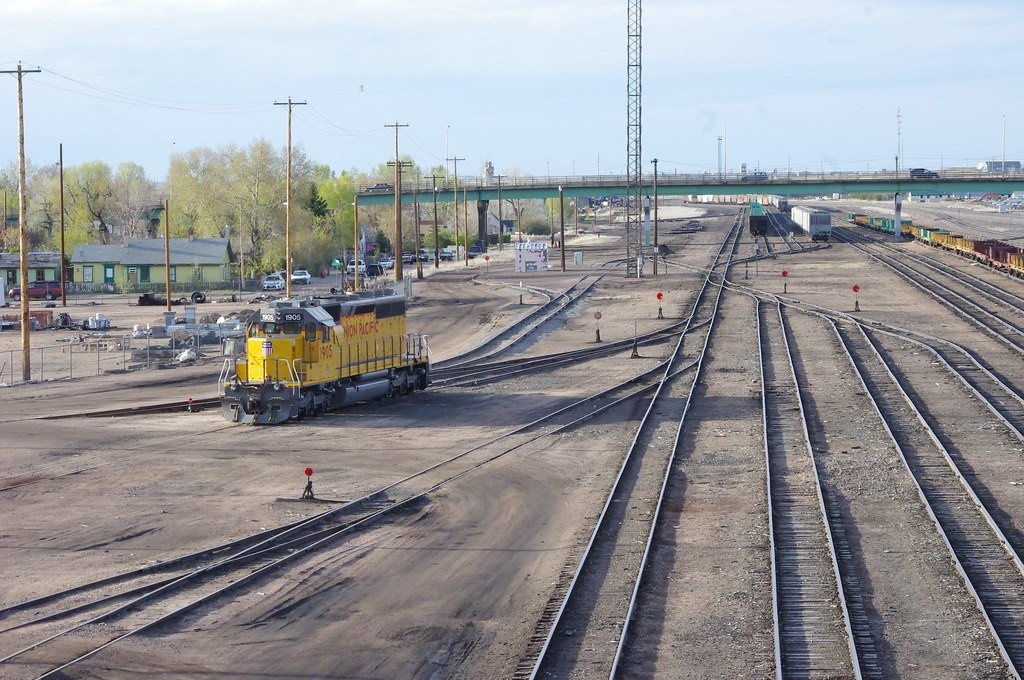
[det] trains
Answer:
[849,213,1024,280]
[218,287,432,426]
[790,205,832,239]
[687,188,788,211]
[749,202,767,235]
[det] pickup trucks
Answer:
[399,252,414,265]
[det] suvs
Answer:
[8,280,64,300]
[366,265,385,278]
[346,259,366,274]
[909,168,940,179]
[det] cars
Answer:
[440,252,453,261]
[377,258,394,269]
[262,274,286,292]
[414,250,428,262]
[468,246,482,258]
[290,270,311,285]
[740,172,768,181]
